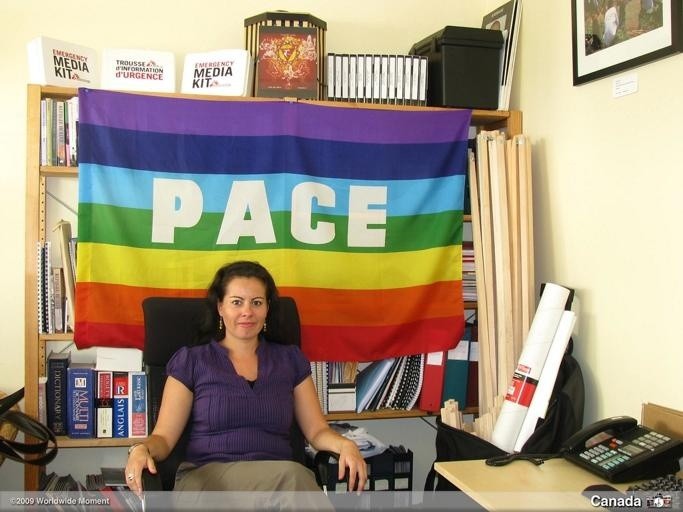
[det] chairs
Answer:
[141,295,361,492]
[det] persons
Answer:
[122,260,370,511]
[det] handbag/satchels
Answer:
[422,389,577,511]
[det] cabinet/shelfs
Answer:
[25,82,524,510]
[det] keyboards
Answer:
[626,475,683,511]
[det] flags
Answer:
[71,84,475,364]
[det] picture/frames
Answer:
[570,0,683,85]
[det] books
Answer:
[459,196,478,303]
[308,308,473,414]
[324,52,430,109]
[39,96,150,512]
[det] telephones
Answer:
[560,415,683,485]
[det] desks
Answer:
[433,455,683,512]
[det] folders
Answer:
[324,444,413,512]
[419,323,478,412]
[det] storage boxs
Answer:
[244,8,502,106]
